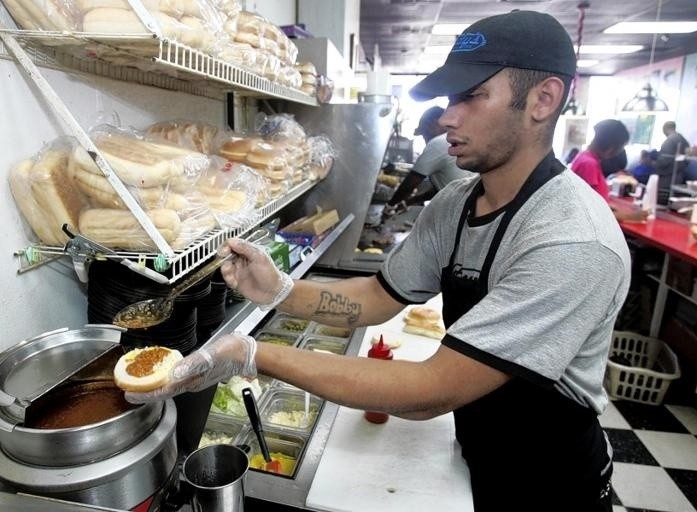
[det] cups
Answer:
[181,443,251,511]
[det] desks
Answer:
[609,192,696,372]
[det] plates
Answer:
[85,245,236,358]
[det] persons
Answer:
[384,106,480,215]
[598,120,697,205]
[124,10,632,512]
[571,118,654,225]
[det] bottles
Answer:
[363,333,394,424]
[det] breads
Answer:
[114,346,182,392]
[404,307,446,339]
[1,1,318,97]
[8,120,310,248]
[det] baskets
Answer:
[605,330,680,407]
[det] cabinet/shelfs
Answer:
[2,1,326,284]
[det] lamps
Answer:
[620,1,668,114]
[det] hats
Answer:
[409,13,577,101]
[414,106,444,134]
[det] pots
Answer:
[0,323,179,508]
